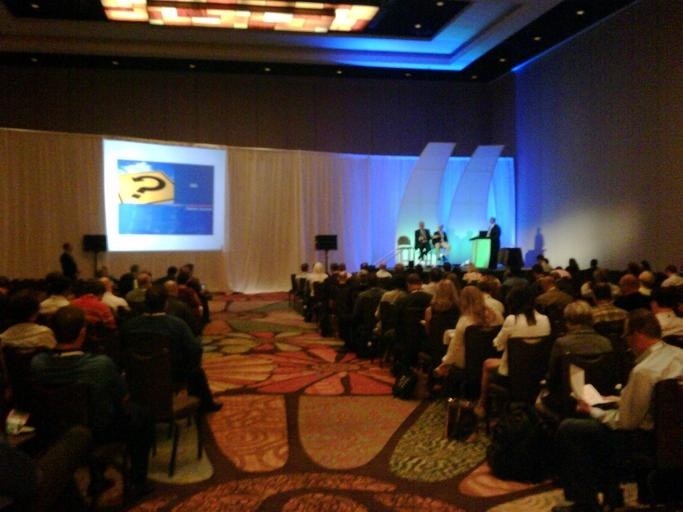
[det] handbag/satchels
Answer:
[444,399,478,439]
[391,371,417,400]
[484,401,557,484]
[410,365,430,400]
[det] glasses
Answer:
[622,328,635,341]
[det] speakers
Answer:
[316,235,337,250]
[84,234,106,251]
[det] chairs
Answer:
[397,235,448,265]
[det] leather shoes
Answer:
[604,487,625,508]
[196,402,221,416]
[122,479,154,503]
[553,503,602,512]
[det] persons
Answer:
[298,256,683,512]
[433,224,449,262]
[1,241,223,512]
[415,220,432,260]
[488,217,501,268]
